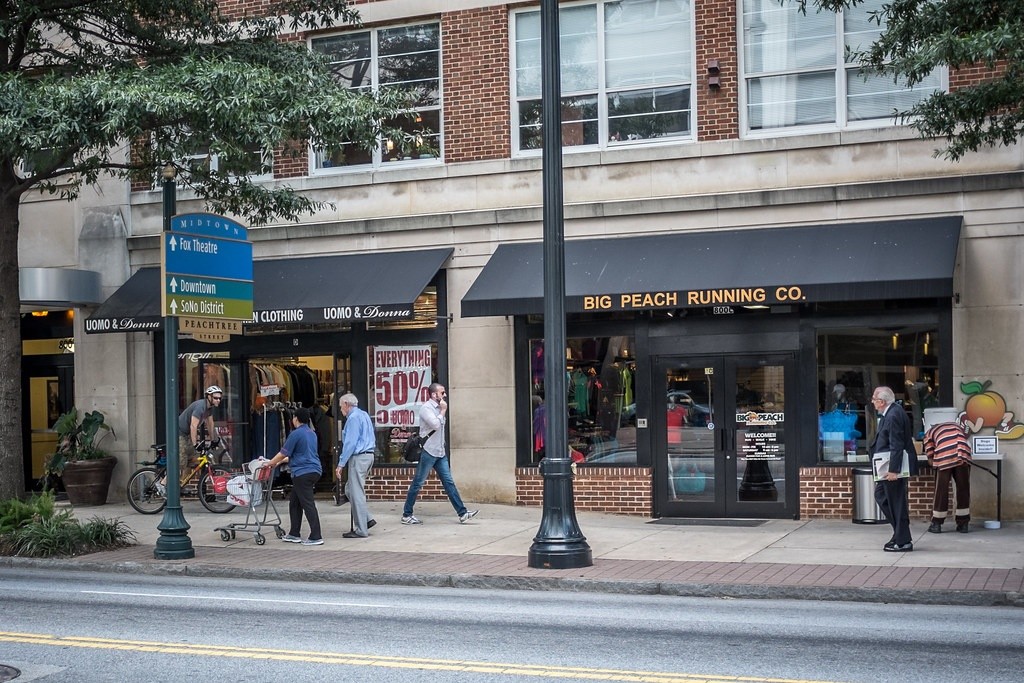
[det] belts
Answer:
[358,452,375,454]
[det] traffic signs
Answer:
[160,231,255,322]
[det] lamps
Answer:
[59,339,74,353]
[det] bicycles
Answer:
[126,440,238,515]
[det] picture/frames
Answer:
[47,379,59,429]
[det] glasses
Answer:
[212,397,222,400]
[441,391,446,395]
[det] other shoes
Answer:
[342,532,360,538]
[367,519,377,529]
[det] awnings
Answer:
[84,248,456,334]
[461,215,962,319]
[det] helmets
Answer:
[205,386,222,395]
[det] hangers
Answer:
[248,360,306,368]
[262,401,302,411]
[573,360,597,375]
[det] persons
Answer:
[267,408,324,545]
[739,380,759,406]
[869,387,917,551]
[401,384,479,525]
[923,423,972,533]
[159,386,222,500]
[335,393,377,538]
[667,389,689,452]
[831,384,857,413]
[375,427,390,463]
[599,357,625,437]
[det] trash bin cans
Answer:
[852,468,891,524]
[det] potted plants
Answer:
[42,408,118,506]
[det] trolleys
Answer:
[211,463,287,545]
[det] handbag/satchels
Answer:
[226,475,262,507]
[402,433,425,462]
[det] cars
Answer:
[623,391,715,425]
[577,429,786,498]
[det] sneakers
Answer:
[459,509,481,524]
[154,480,167,498]
[928,523,941,533]
[883,542,913,552]
[401,516,423,525]
[301,538,324,546]
[956,523,968,533]
[283,535,302,543]
